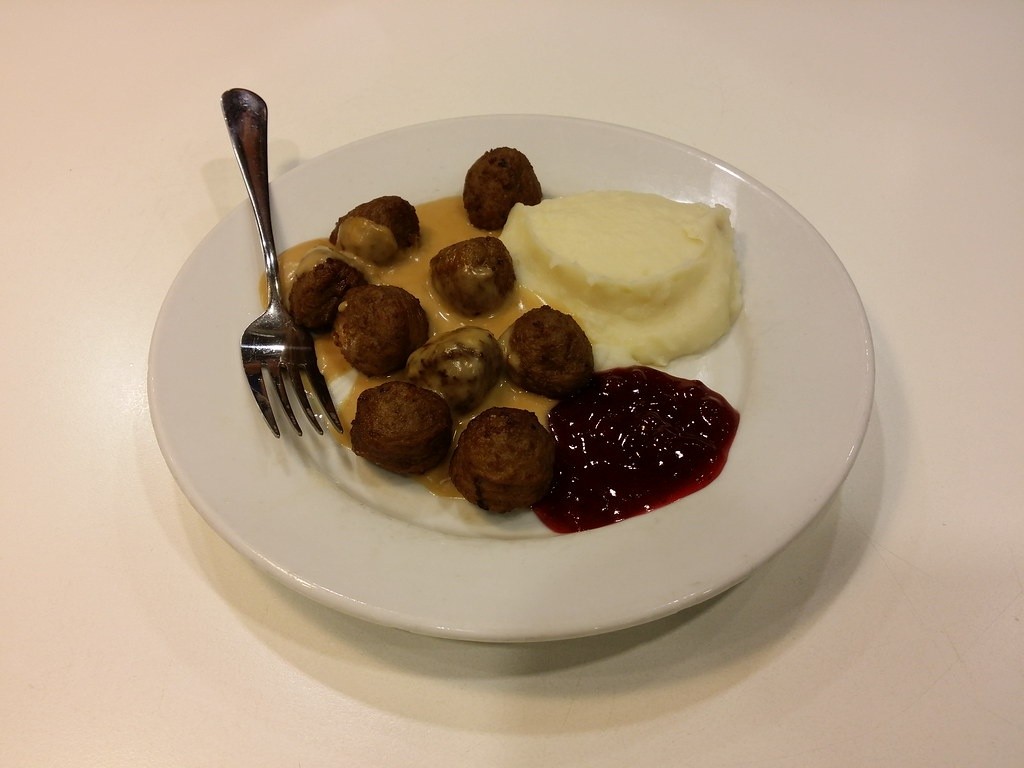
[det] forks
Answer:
[220,87,347,441]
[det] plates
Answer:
[146,112,878,650]
